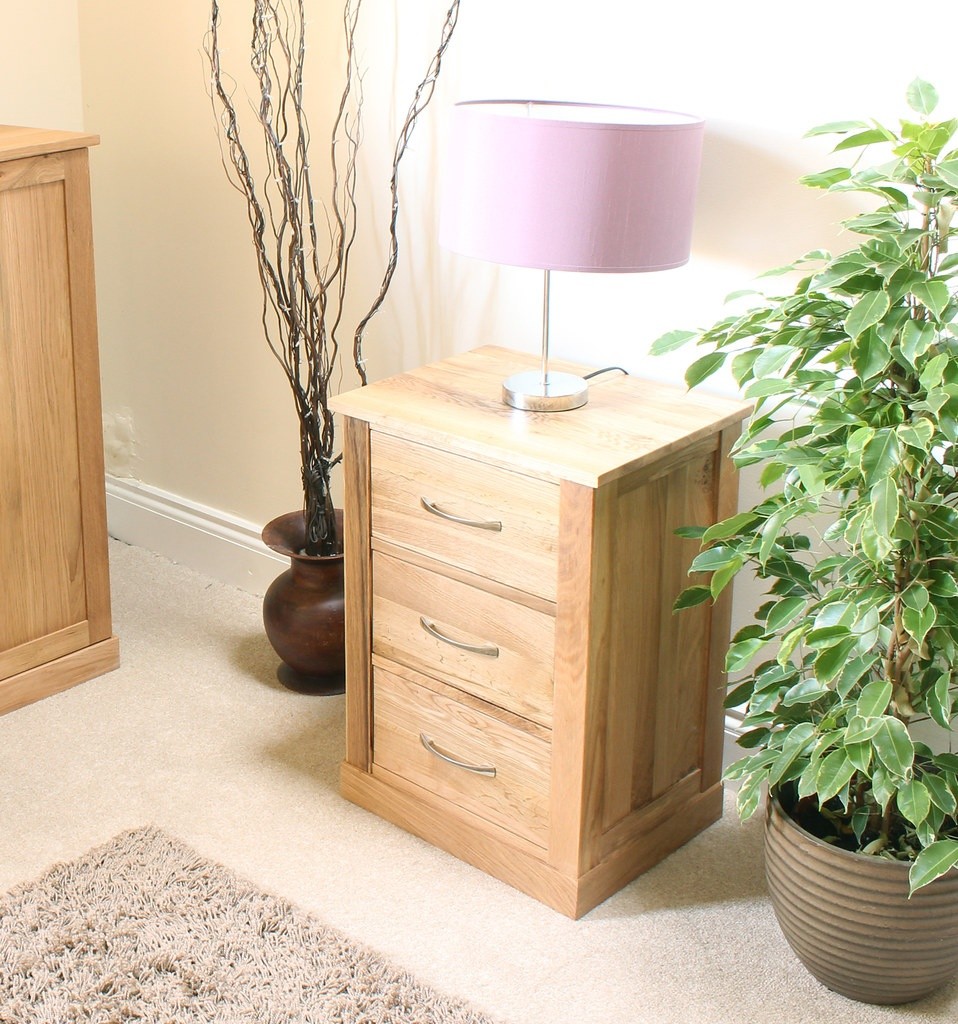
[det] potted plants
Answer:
[642,74,958,1009]
[199,2,462,695]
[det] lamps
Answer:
[436,98,704,412]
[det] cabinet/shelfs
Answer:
[0,122,119,715]
[325,344,753,918]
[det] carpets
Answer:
[0,822,500,1024]
